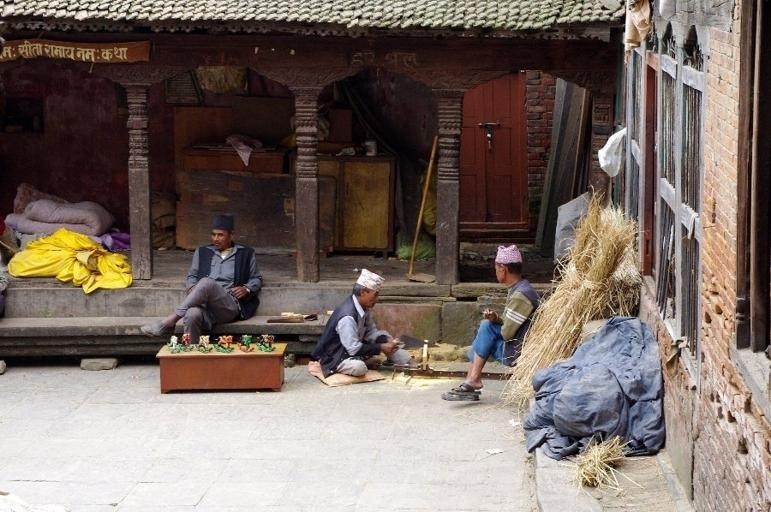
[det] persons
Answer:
[140,213,263,343]
[441,245,542,401]
[310,268,431,377]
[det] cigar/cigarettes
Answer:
[479,311,493,316]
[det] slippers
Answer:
[450,383,481,395]
[442,391,479,400]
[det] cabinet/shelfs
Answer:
[289,155,395,252]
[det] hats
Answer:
[356,268,385,292]
[494,245,522,264]
[213,213,232,230]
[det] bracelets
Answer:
[243,285,250,294]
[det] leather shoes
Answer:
[141,321,176,338]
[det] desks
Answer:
[156,342,287,393]
